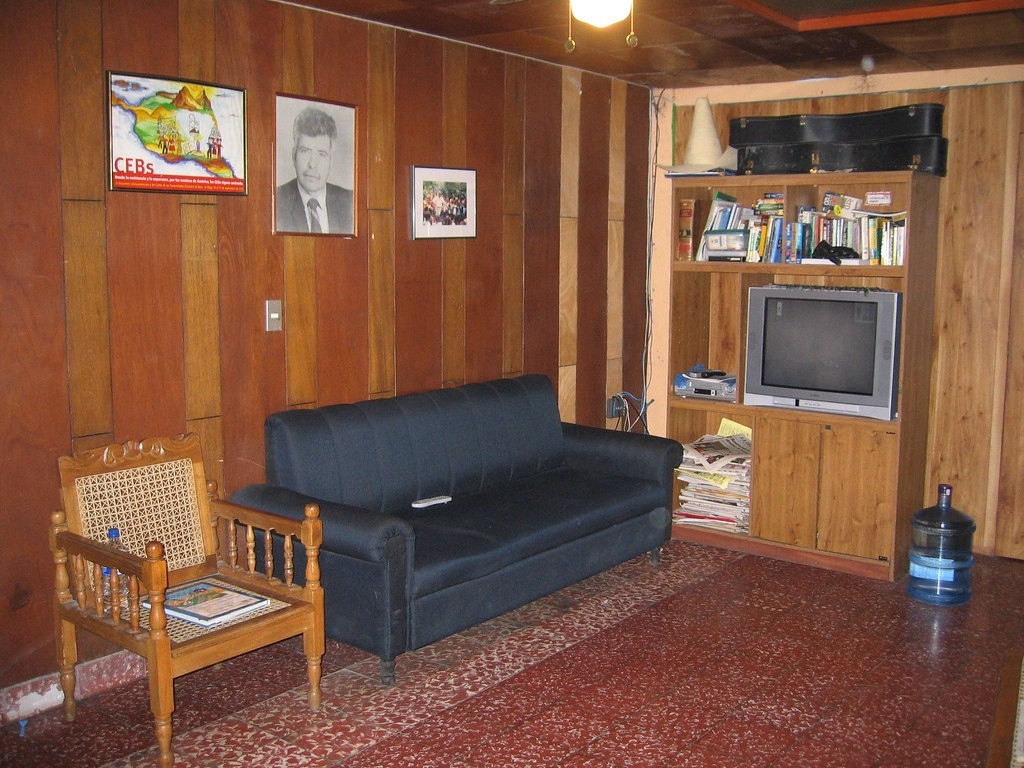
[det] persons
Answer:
[425,185,468,225]
[278,108,354,234]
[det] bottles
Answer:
[907,484,976,606]
[101,528,128,614]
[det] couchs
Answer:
[232,375,684,687]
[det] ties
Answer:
[307,198,323,235]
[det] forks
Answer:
[19,719,28,737]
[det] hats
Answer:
[654,97,737,172]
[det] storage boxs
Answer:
[704,229,751,251]
[678,199,700,261]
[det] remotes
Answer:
[690,370,722,378]
[701,371,726,378]
[411,496,452,508]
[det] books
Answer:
[141,581,270,626]
[678,193,906,267]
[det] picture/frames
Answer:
[410,165,477,241]
[105,69,248,197]
[271,92,360,239]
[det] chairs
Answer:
[49,432,325,768]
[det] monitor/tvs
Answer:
[743,287,903,422]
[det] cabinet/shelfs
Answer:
[664,170,942,581]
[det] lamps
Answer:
[564,0,639,53]
